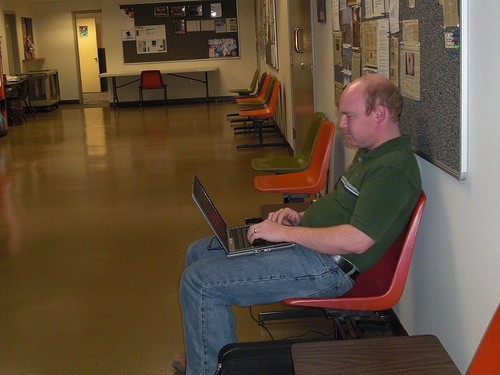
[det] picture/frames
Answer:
[171,5,186,18]
[173,19,186,35]
[188,5,203,17]
[154,6,168,18]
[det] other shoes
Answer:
[171,353,186,373]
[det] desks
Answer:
[218,335,462,375]
[5,78,35,87]
[99,67,219,110]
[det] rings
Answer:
[254,228,257,233]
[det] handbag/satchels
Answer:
[213,337,317,375]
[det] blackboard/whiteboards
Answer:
[119,0,241,65]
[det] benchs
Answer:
[252,112,335,203]
[259,190,426,340]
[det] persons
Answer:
[171,72,422,375]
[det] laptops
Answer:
[191,173,297,258]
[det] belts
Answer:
[326,254,361,280]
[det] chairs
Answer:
[139,70,168,107]
[6,79,38,121]
[226,70,287,151]
